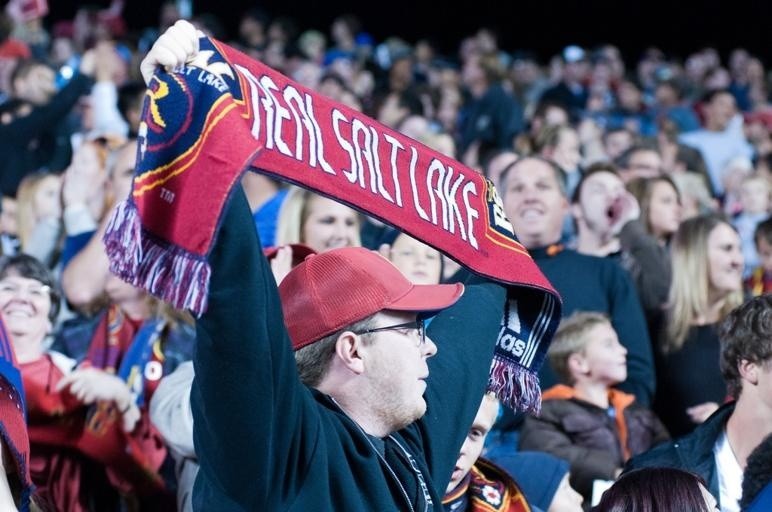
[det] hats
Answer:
[277,246,465,351]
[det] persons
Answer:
[0,0,771,511]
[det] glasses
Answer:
[330,313,426,354]
